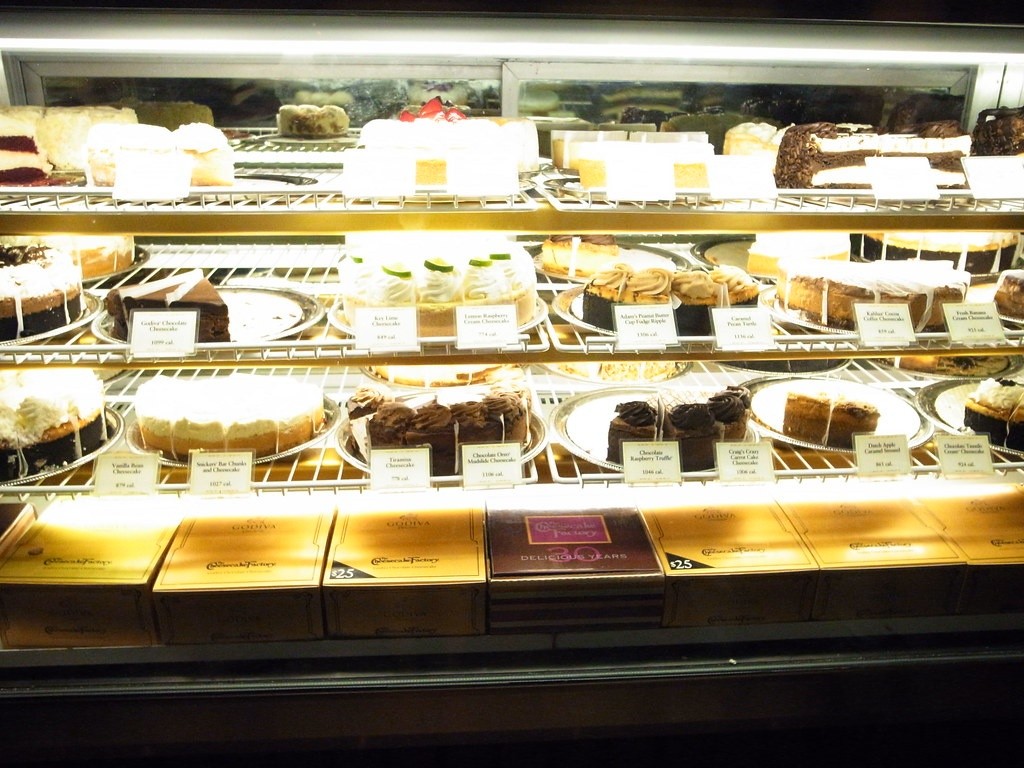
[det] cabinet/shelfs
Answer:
[0,195,1024,757]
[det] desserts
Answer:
[1,100,1024,468]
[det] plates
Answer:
[114,173,1023,480]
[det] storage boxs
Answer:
[782,499,971,620]
[483,509,664,638]
[639,509,821,621]
[146,503,334,641]
[0,496,177,648]
[323,506,488,639]
[905,491,1024,610]
